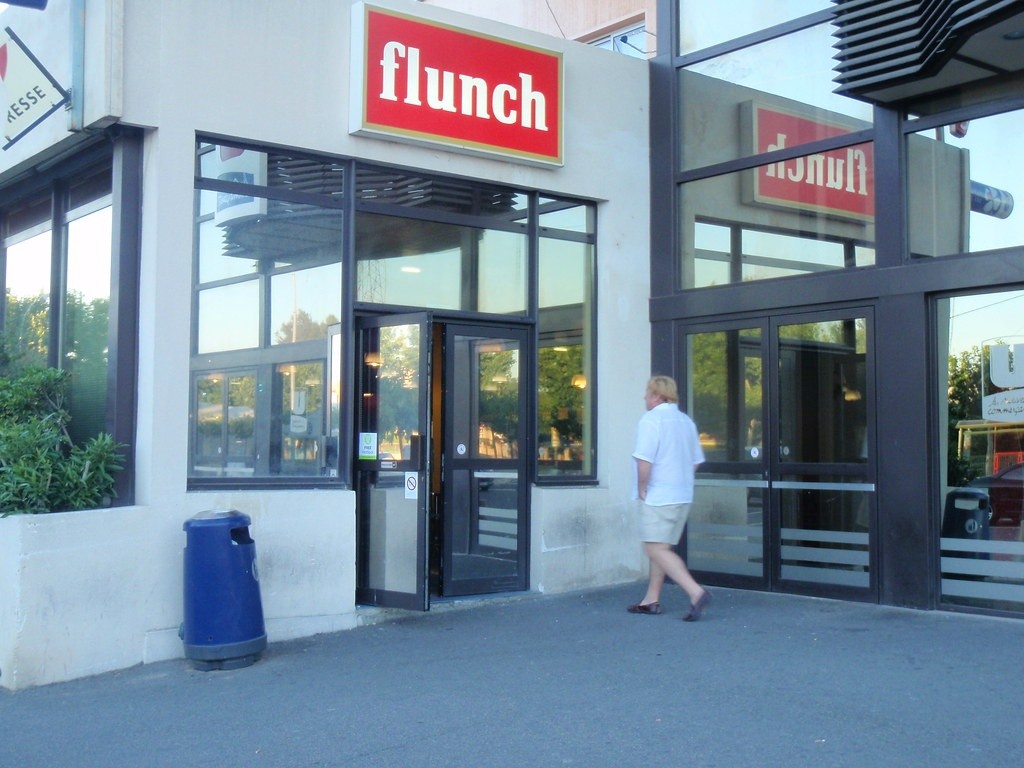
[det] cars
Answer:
[965,461,1024,526]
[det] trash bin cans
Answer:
[178,508,268,672]
[940,488,992,582]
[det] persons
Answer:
[626,375,713,622]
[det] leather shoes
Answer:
[627,602,661,614]
[683,588,713,622]
